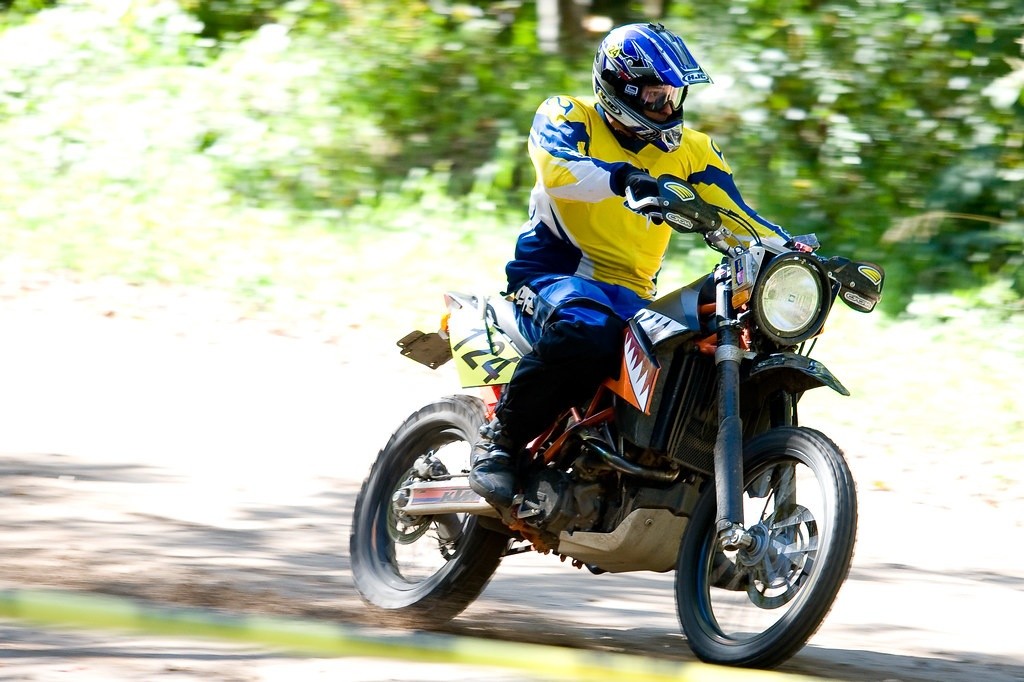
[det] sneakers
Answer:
[469,416,516,507]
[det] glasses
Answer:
[609,75,689,112]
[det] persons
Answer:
[471,21,827,507]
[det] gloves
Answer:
[622,171,664,227]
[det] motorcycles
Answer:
[349,174,885,668]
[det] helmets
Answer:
[591,22,714,153]
